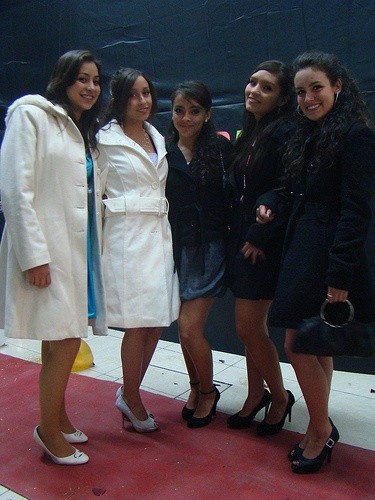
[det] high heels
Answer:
[116,395,158,432]
[288,417,333,461]
[227,389,271,429]
[60,426,88,443]
[187,384,220,428]
[256,390,295,435]
[291,423,339,474]
[115,386,154,419]
[33,425,89,465]
[182,381,200,420]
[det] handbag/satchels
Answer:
[290,299,375,359]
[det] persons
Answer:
[85,67,181,433]
[164,79,241,429]
[254,52,374,474]
[0,48,108,466]
[225,58,295,435]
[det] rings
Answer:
[327,295,333,298]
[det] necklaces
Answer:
[123,128,155,152]
